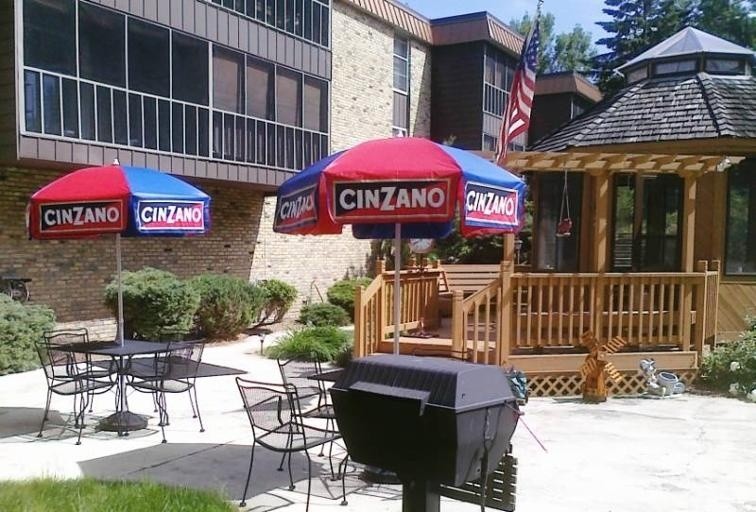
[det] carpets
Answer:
[90,355,246,379]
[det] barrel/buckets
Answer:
[657,372,678,396]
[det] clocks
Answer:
[406,236,436,253]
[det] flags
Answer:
[494,15,542,162]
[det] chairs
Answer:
[234,376,350,512]
[30,325,209,444]
[277,349,338,472]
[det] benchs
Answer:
[431,265,529,315]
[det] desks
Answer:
[307,369,376,488]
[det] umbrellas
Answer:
[24,156,214,430]
[272,131,529,485]
